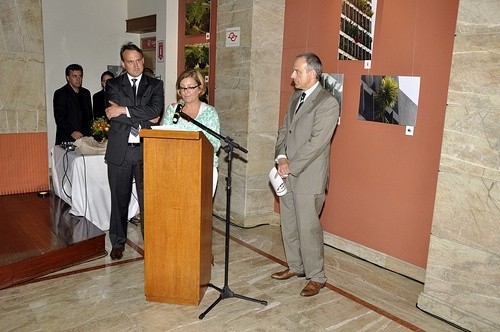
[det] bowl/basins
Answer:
[62,142,75,151]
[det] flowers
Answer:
[92,117,109,131]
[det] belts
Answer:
[126,143,141,149]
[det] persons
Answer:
[160,68,222,265]
[53,64,93,208]
[93,71,115,126]
[271,52,340,297]
[142,68,164,126]
[104,42,165,260]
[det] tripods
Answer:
[181,117,266,318]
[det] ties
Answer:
[130,78,140,137]
[295,93,307,116]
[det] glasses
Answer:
[179,85,201,92]
[103,80,107,83]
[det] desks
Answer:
[50,145,143,232]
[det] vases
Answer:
[91,131,103,143]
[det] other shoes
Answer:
[110,245,125,260]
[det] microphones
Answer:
[172,99,185,124]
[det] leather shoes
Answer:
[301,278,327,297]
[270,268,306,279]
